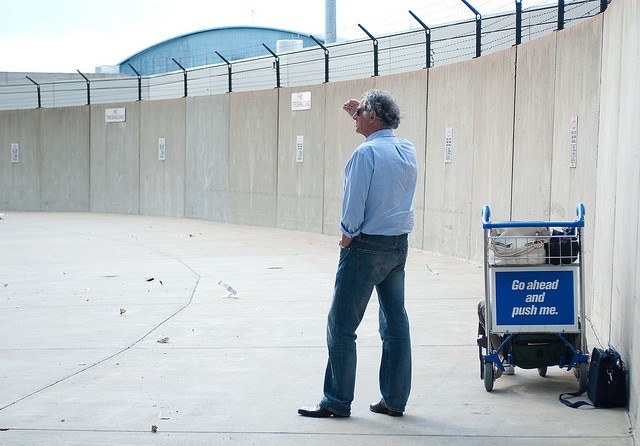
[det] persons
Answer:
[297,89,418,418]
[477,299,515,376]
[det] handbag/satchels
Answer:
[544,227,578,265]
[559,348,629,408]
[488,220,550,266]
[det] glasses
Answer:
[355,107,369,116]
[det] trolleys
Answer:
[476,203,590,393]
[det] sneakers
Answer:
[493,356,514,375]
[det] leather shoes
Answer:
[298,402,351,418]
[369,399,404,417]
